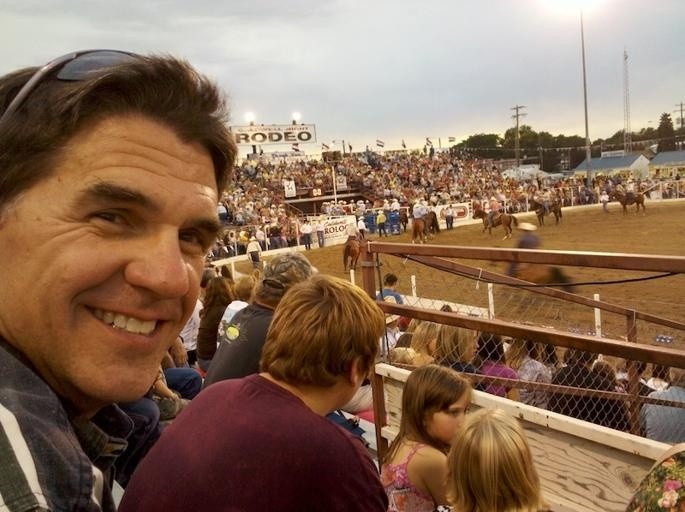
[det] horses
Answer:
[529,198,563,227]
[342,228,366,274]
[472,206,517,241]
[609,188,645,216]
[411,211,441,245]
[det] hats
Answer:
[516,223,537,231]
[219,188,455,223]
[262,250,320,287]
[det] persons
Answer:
[0,47,236,512]
[203,147,684,259]
[88,238,685,511]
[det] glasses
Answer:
[0,47,156,135]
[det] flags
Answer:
[439,138,441,149]
[321,142,330,150]
[448,137,456,142]
[402,139,406,147]
[377,138,385,148]
[426,138,433,146]
